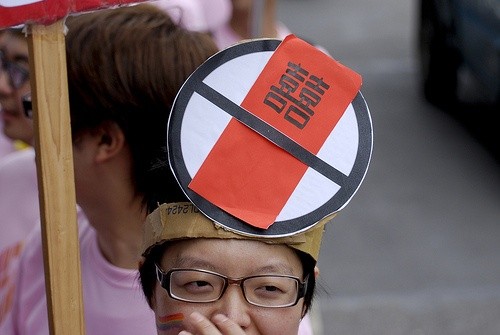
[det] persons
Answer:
[0,4,314,335]
[0,28,41,326]
[138,152,325,335]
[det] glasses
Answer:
[0,47,33,88]
[21,93,37,120]
[152,264,310,309]
